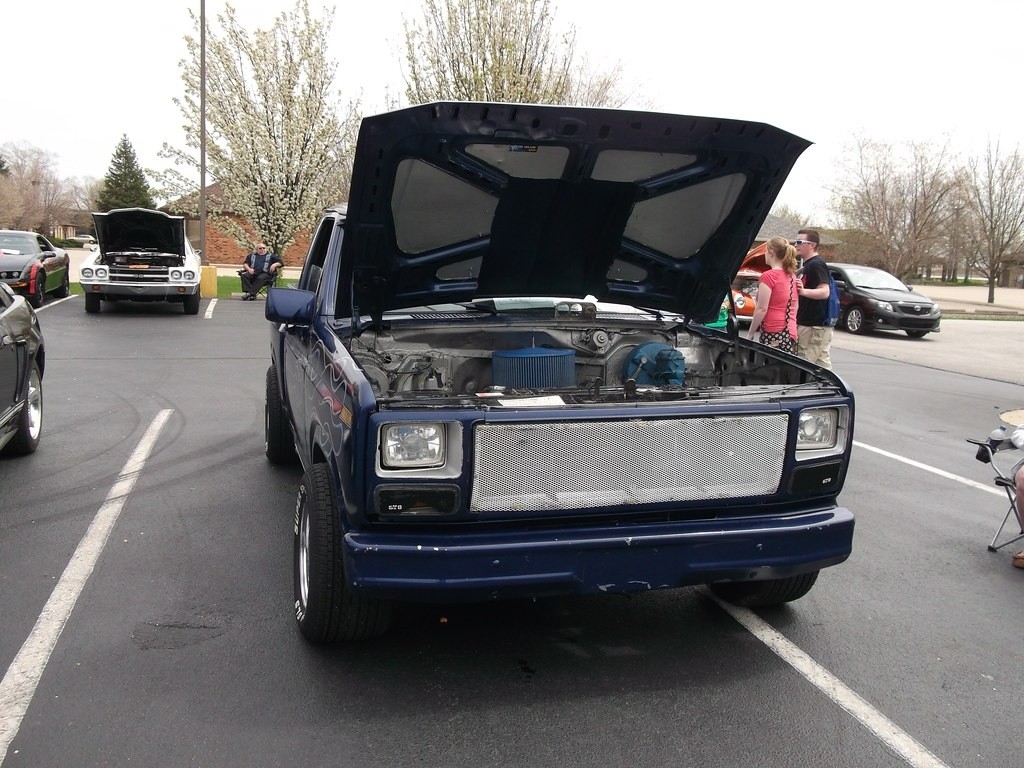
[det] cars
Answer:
[79,208,202,314]
[260,96,862,651]
[1,283,46,458]
[814,262,942,339]
[66,235,96,243]
[726,243,767,326]
[1,230,71,308]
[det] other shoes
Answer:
[1012,552,1024,569]
[242,292,256,301]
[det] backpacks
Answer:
[818,275,841,326]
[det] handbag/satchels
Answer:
[759,327,793,354]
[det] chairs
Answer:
[966,409,1024,552]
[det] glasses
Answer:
[795,239,813,245]
[258,247,265,249]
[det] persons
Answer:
[1010,422,1023,570]
[744,237,800,356]
[796,229,834,372]
[240,243,285,302]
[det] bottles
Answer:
[975,425,1007,464]
